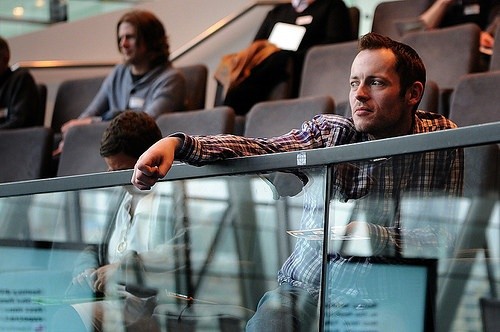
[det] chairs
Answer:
[0,0,500,183]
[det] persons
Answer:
[42,109,214,332]
[222,0,353,115]
[42,8,187,179]
[395,0,500,75]
[0,36,41,131]
[130,32,463,332]
